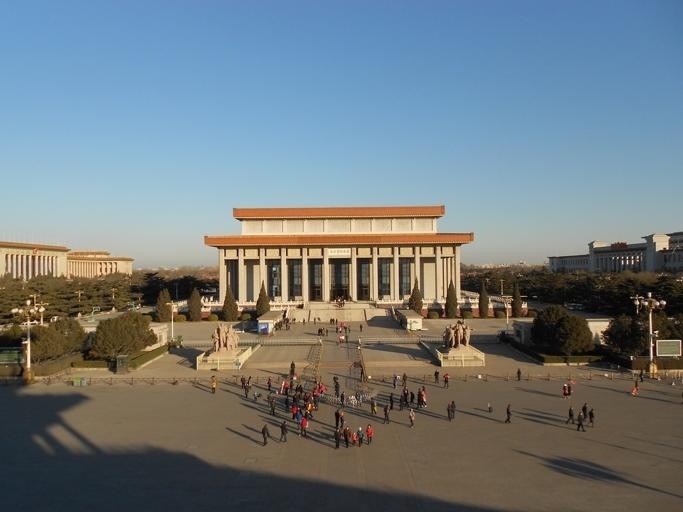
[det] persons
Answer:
[359,324,362,332]
[447,403,453,421]
[239,369,426,448]
[639,370,643,381]
[444,374,448,389]
[562,383,568,399]
[317,317,349,344]
[211,375,216,384]
[582,402,587,418]
[210,380,216,393]
[588,408,594,423]
[516,368,520,381]
[504,403,511,422]
[450,400,456,416]
[433,368,439,383]
[576,412,586,431]
[567,383,571,396]
[567,406,574,423]
[211,323,238,351]
[442,319,475,348]
[335,295,344,307]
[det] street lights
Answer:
[166,300,179,340]
[111,288,117,312]
[10,293,49,384]
[630,292,666,378]
[500,279,506,296]
[74,290,85,318]
[501,296,514,327]
